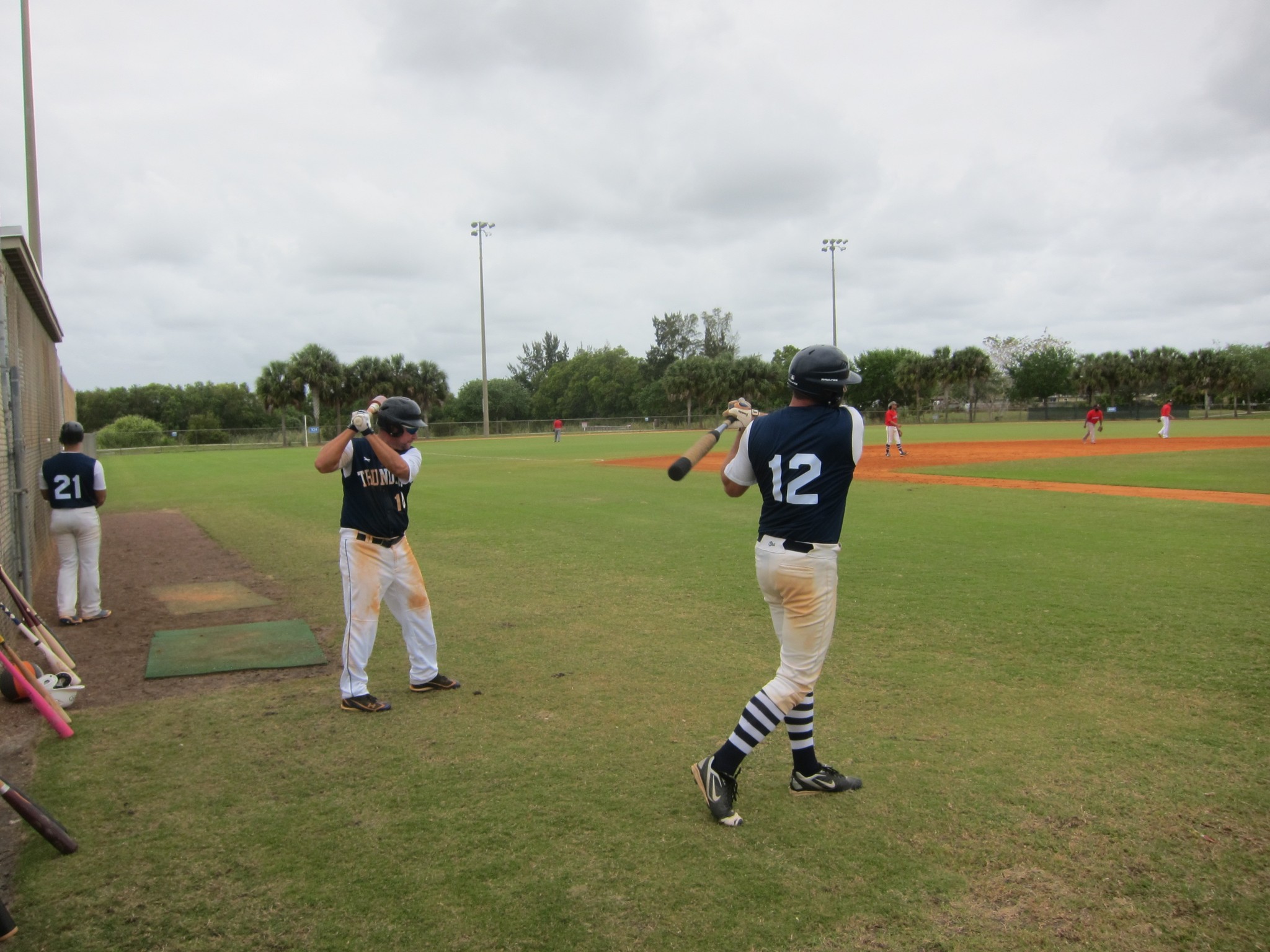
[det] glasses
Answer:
[402,425,418,434]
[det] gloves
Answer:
[353,410,375,435]
[722,397,759,429]
[347,410,359,432]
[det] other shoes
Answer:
[0,904,18,940]
[900,452,909,457]
[1082,439,1087,443]
[885,453,892,457]
[1091,441,1096,444]
[1158,433,1161,438]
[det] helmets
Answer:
[38,672,85,708]
[377,396,429,436]
[787,344,862,407]
[59,421,85,442]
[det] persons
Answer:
[885,401,909,457]
[1157,400,1175,439]
[40,420,111,626]
[691,345,865,828]
[1082,404,1103,444]
[315,396,462,714]
[553,418,562,442]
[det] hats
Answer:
[1167,400,1173,402]
[1094,404,1100,408]
[890,401,899,406]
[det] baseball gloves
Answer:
[898,430,902,437]
[1158,418,1161,422]
[1098,426,1103,432]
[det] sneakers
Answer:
[59,615,82,626]
[82,610,111,622]
[788,762,863,797]
[690,754,742,827]
[409,673,461,691]
[341,694,391,713]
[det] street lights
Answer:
[821,238,849,347]
[471,221,495,437]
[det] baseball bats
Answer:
[0,635,72,724]
[0,651,74,739]
[668,415,739,481]
[0,899,19,941]
[0,775,78,855]
[0,564,76,671]
[352,395,388,426]
[0,602,81,686]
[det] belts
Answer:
[357,533,403,548]
[757,533,814,553]
